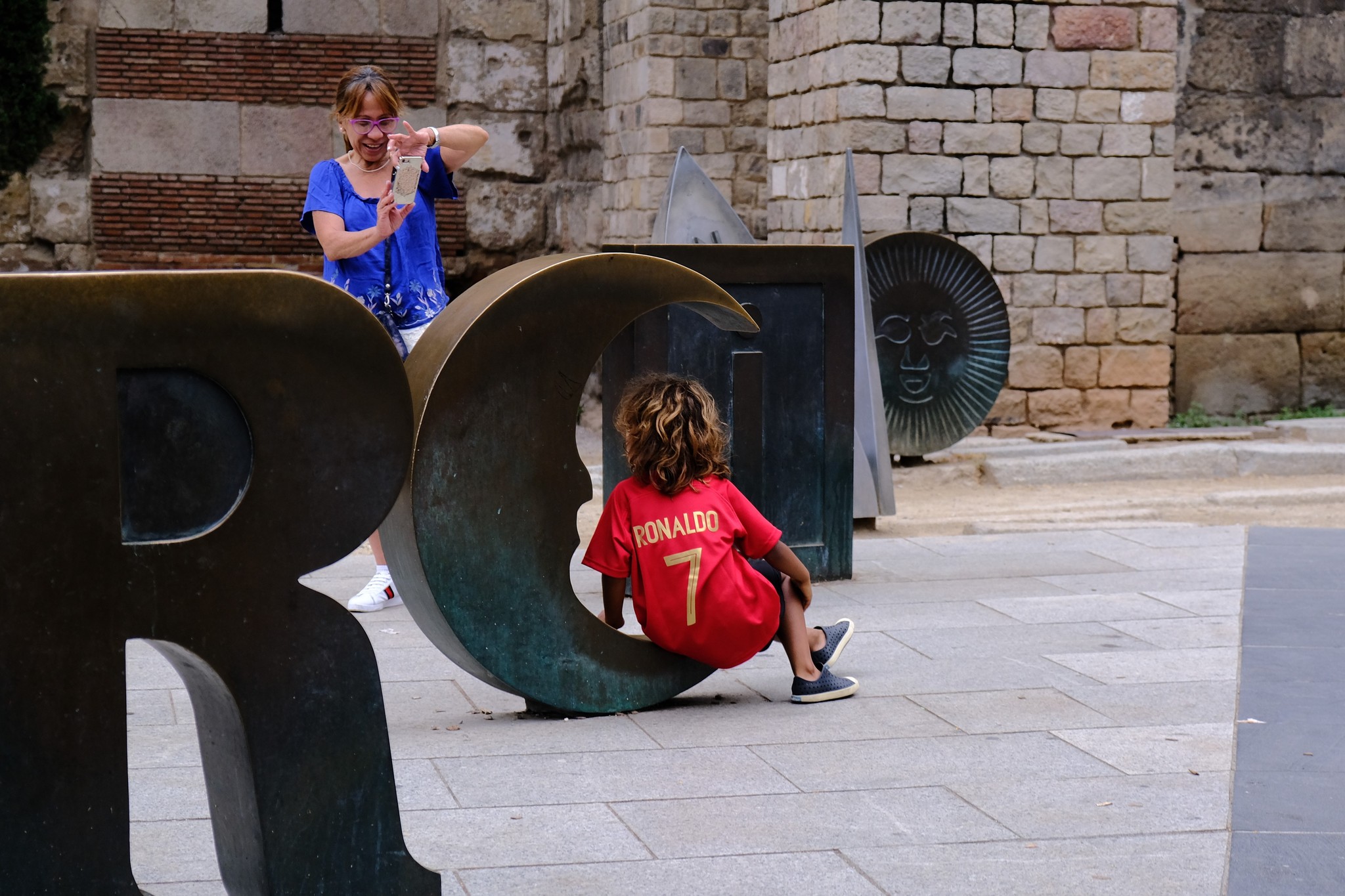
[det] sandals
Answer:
[791,661,859,702]
[811,617,854,672]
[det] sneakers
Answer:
[347,570,404,613]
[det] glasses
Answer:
[339,113,399,135]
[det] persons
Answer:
[301,67,488,612]
[580,377,858,703]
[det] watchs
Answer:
[426,127,440,149]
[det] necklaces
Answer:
[348,150,391,172]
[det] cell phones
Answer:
[391,157,423,205]
